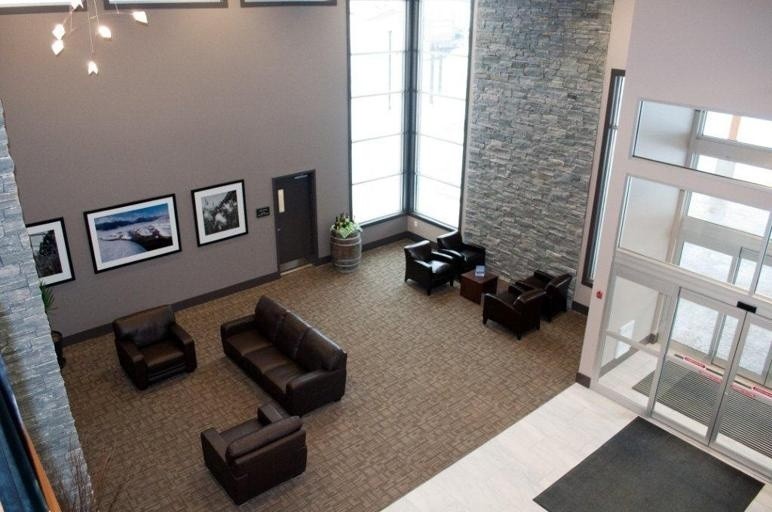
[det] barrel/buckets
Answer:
[330,225,362,273]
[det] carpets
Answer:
[533,415,766,512]
[632,354,772,460]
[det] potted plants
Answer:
[39,279,66,369]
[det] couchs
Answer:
[404,240,455,296]
[202,404,306,505]
[517,270,572,322]
[436,231,486,282]
[113,305,198,391]
[483,283,546,339]
[220,295,346,417]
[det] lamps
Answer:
[51,1,148,76]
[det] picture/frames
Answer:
[191,180,248,248]
[84,193,183,273]
[25,216,76,289]
